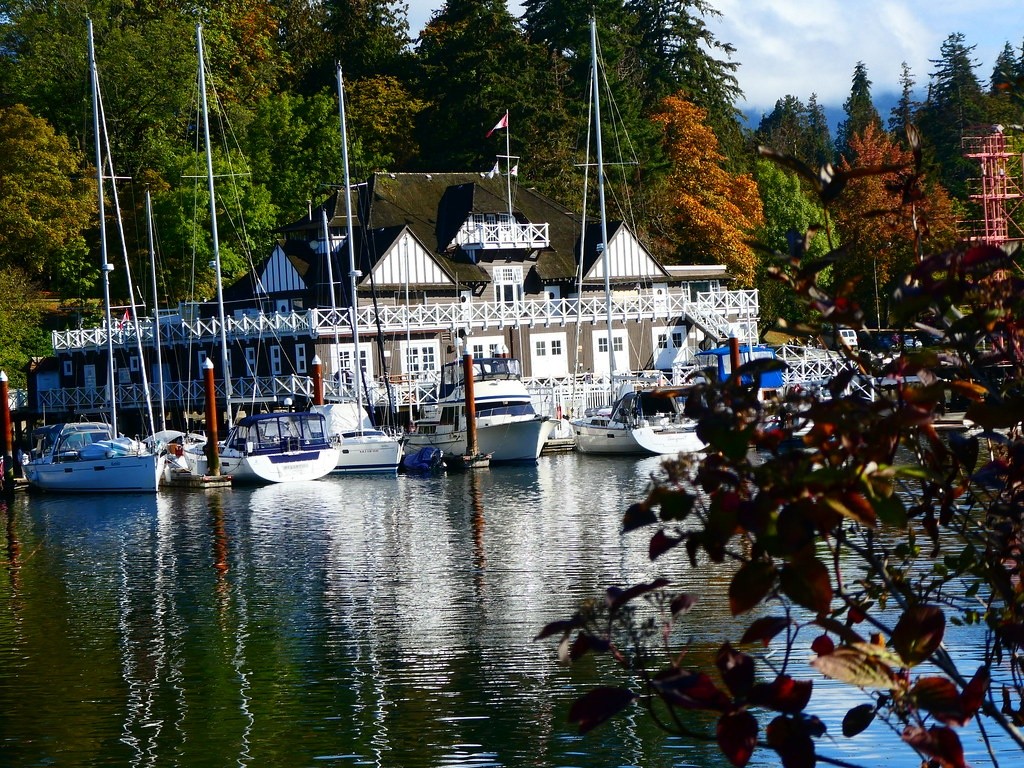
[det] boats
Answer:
[394,271,563,464]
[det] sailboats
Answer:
[562,13,711,457]
[17,16,411,492]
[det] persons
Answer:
[788,335,814,354]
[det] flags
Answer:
[120,311,129,329]
[486,114,508,137]
